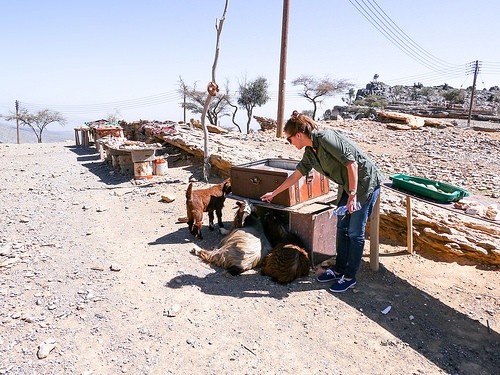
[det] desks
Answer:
[370,182,500,271]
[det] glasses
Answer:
[287,133,296,143]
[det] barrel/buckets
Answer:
[134,161,150,176]
[152,160,168,175]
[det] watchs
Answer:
[349,191,358,196]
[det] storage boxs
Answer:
[230,158,338,268]
[97,143,155,179]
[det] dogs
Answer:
[186,198,313,287]
[185,176,233,242]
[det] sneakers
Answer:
[317,268,344,282]
[328,275,356,293]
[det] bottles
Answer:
[333,202,362,218]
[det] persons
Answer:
[260,110,385,293]
